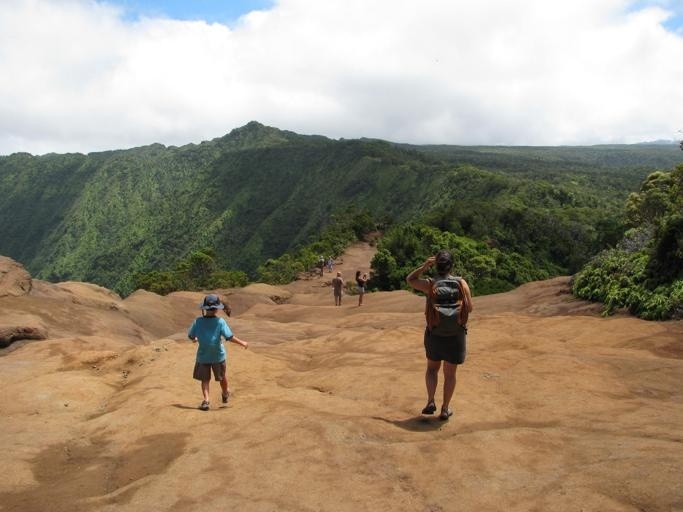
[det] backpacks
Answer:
[428,276,465,341]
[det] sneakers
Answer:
[223,388,230,403]
[200,400,209,410]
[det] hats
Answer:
[199,294,224,310]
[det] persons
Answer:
[318,256,324,277]
[187,294,247,410]
[332,272,344,305]
[356,271,367,306]
[327,256,333,272]
[407,251,473,420]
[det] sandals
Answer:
[422,400,437,415]
[439,407,452,420]
